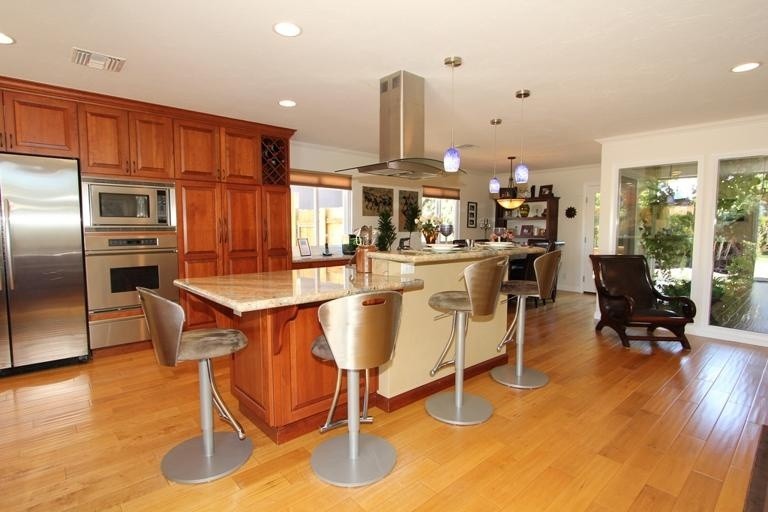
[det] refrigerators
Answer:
[0,150,90,374]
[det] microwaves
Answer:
[89,188,172,225]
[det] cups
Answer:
[356,244,376,271]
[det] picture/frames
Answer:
[297,237,311,258]
[467,201,477,229]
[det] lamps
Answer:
[444,56,530,209]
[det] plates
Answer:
[486,242,513,247]
[423,243,461,253]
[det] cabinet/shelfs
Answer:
[76,99,174,180]
[172,118,264,185]
[494,200,560,244]
[1,76,79,160]
[174,179,262,328]
[258,183,294,272]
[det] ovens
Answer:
[84,231,178,317]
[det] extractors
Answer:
[333,68,469,182]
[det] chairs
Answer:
[304,290,412,487]
[133,286,253,483]
[491,250,564,388]
[422,251,498,425]
[590,254,693,357]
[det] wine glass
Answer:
[440,225,453,246]
[493,227,505,244]
